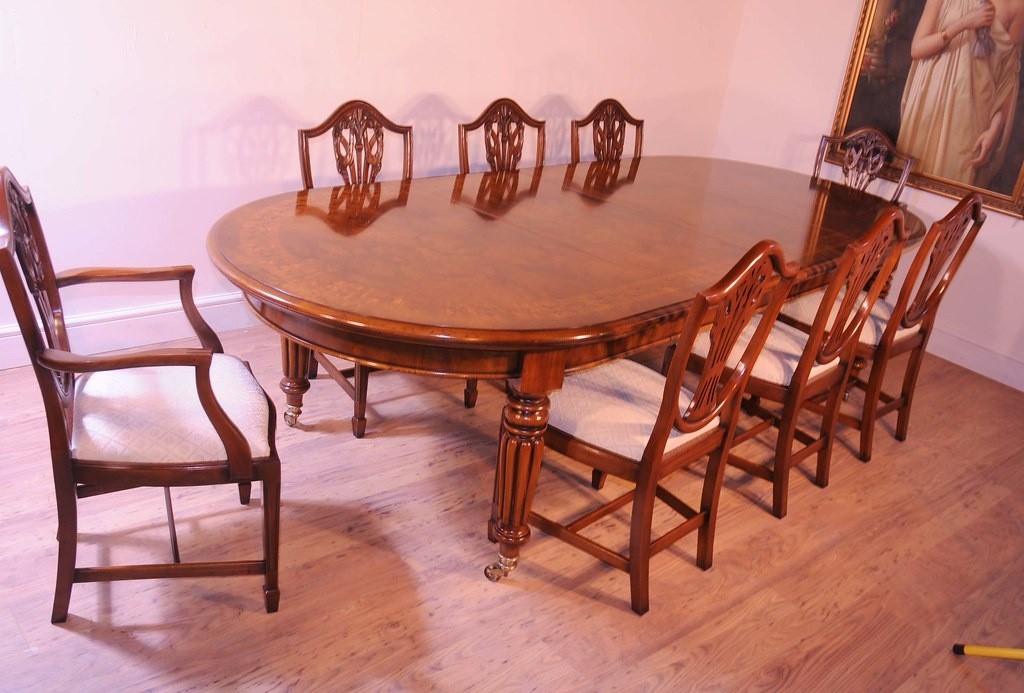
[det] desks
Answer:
[207,158,926,582]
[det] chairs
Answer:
[777,191,988,463]
[506,237,799,617]
[661,207,911,520]
[0,165,284,627]
[570,96,645,161]
[295,97,478,440]
[459,97,547,175]
[812,126,915,200]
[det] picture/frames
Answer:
[825,0,1024,222]
